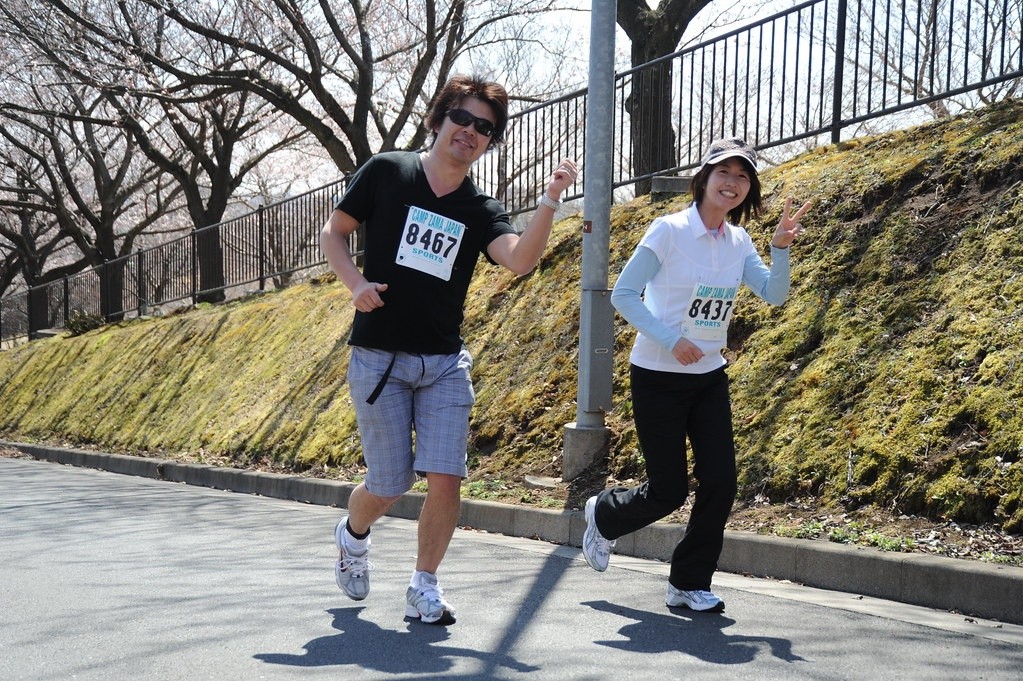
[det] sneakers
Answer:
[666,579,725,612]
[405,572,456,625]
[582,495,617,572]
[335,515,374,601]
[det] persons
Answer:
[319,78,577,625]
[582,138,812,612]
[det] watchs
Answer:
[542,193,563,211]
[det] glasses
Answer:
[444,108,495,137]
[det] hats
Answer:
[700,137,759,176]
